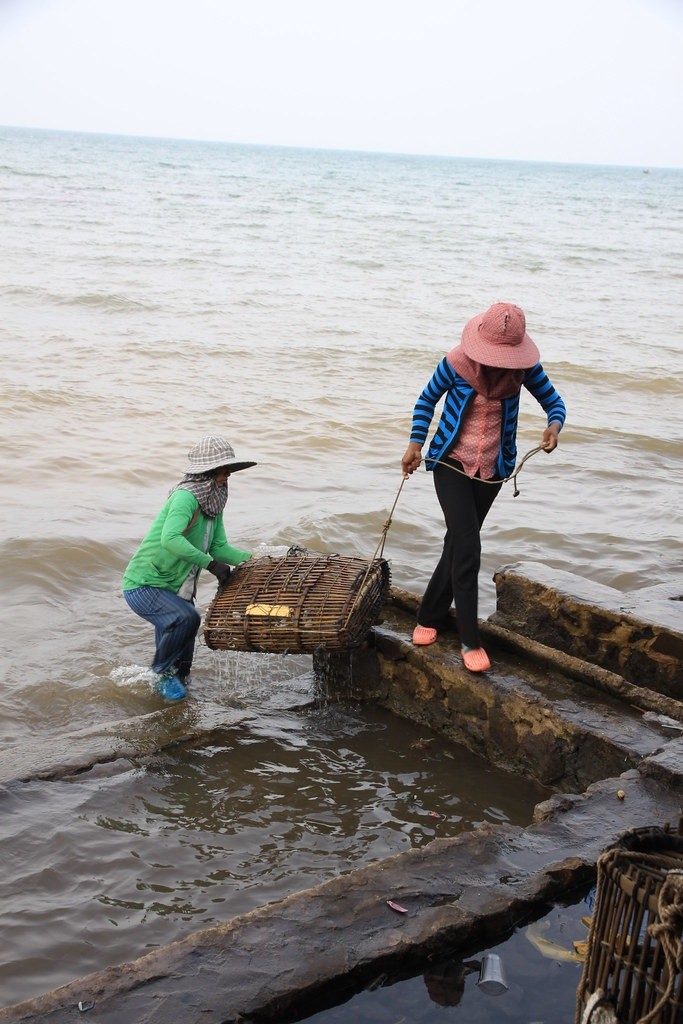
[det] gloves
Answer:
[206,560,231,586]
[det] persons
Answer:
[123,437,257,701]
[401,302,566,669]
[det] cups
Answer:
[477,953,509,991]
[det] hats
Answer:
[178,435,256,474]
[461,302,539,370]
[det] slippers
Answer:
[461,647,490,672]
[413,626,437,645]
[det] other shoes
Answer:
[158,674,185,700]
